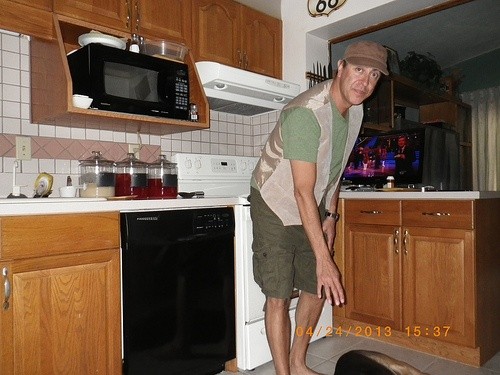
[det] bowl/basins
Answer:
[139,39,188,62]
[72,94,93,109]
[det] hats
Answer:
[343,40,390,76]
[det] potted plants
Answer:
[400,53,447,96]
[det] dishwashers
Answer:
[119,207,236,375]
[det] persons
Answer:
[394,136,416,174]
[250,40,389,375]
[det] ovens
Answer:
[233,205,333,371]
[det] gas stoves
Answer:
[172,154,259,204]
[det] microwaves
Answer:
[67,42,189,120]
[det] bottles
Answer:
[386,176,394,188]
[78,151,178,200]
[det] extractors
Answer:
[195,61,302,118]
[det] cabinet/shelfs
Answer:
[345,199,476,350]
[0,0,283,79]
[361,75,472,147]
[0,212,122,375]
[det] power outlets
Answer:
[15,136,31,160]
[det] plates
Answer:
[78,32,126,50]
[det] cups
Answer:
[59,186,76,197]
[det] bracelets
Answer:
[325,212,338,220]
